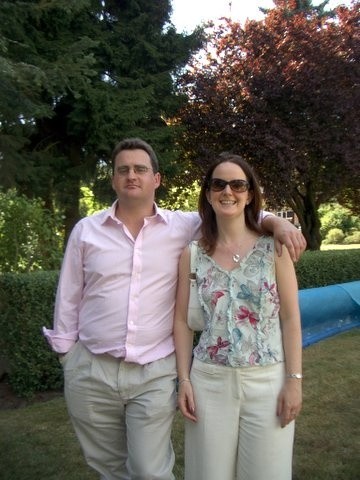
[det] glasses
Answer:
[113,165,157,175]
[207,178,251,193]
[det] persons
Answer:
[173,154,302,480]
[42,138,306,480]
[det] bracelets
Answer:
[287,374,302,379]
[177,378,191,385]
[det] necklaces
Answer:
[217,238,249,262]
